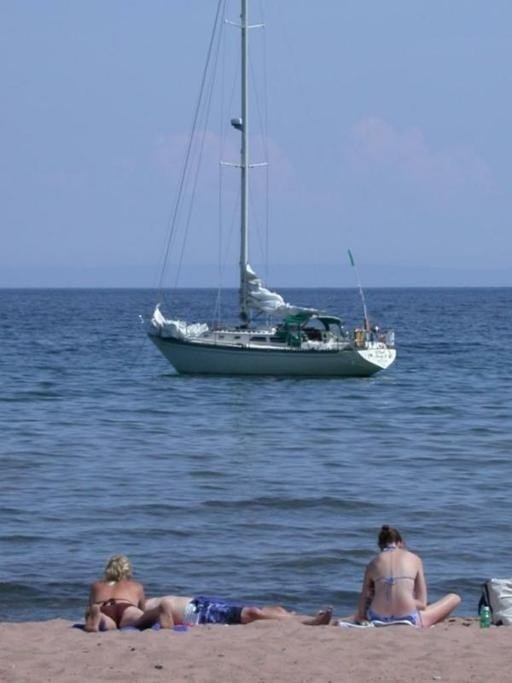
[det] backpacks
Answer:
[478,578,512,625]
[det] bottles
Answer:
[480,606,491,628]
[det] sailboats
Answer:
[132,0,400,379]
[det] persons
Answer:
[146,596,333,626]
[84,553,174,632]
[351,524,462,631]
